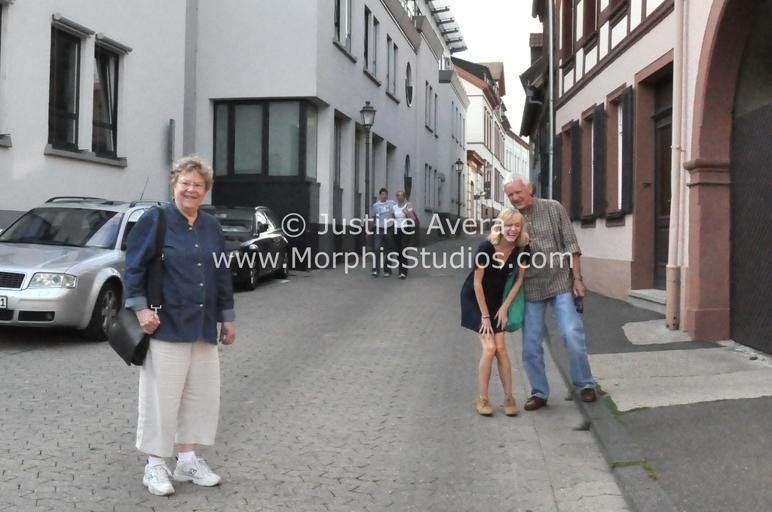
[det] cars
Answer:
[198,204,291,292]
[0,196,171,343]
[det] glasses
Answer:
[574,296,583,313]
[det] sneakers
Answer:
[477,395,493,416]
[172,456,222,488]
[370,271,407,280]
[142,463,176,497]
[503,398,518,416]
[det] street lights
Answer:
[359,100,377,261]
[455,158,464,235]
[475,191,487,223]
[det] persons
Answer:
[502,172,597,410]
[393,190,413,278]
[368,188,415,278]
[124,156,236,496]
[460,208,531,416]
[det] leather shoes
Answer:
[580,389,597,403]
[524,395,548,411]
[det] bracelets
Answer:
[572,276,582,280]
[482,315,490,319]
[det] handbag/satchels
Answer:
[500,244,527,333]
[405,204,419,231]
[105,205,164,368]
